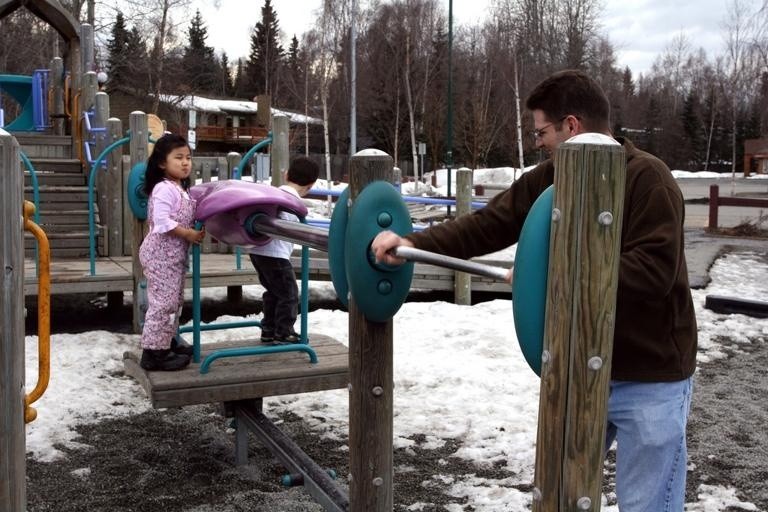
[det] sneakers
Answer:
[261,325,309,345]
[139,339,194,371]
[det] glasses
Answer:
[529,123,551,137]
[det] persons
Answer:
[247,155,320,344]
[139,134,206,371]
[370,68,698,510]
